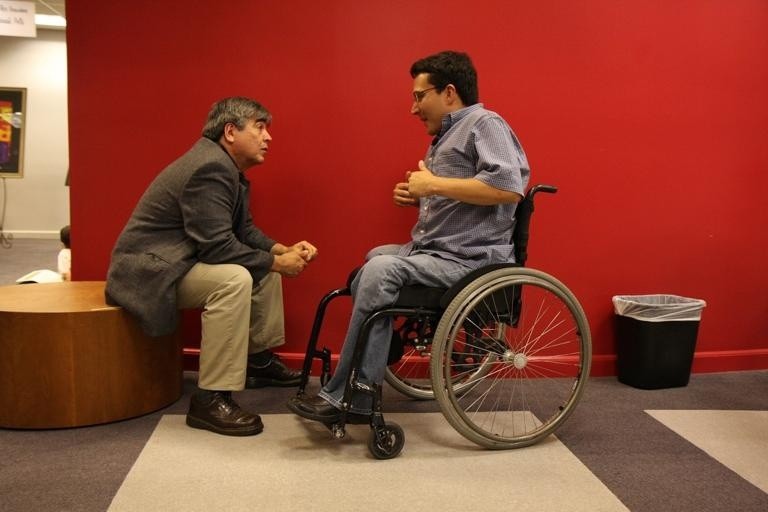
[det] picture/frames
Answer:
[0,86,26,180]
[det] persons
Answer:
[17,224,72,286]
[104,96,319,437]
[285,50,530,425]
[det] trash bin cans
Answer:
[613,294,706,389]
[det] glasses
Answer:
[413,86,440,103]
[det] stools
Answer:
[0,281,183,431]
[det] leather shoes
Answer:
[286,395,374,424]
[245,354,310,389]
[186,392,264,435]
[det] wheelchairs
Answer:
[287,183,591,460]
